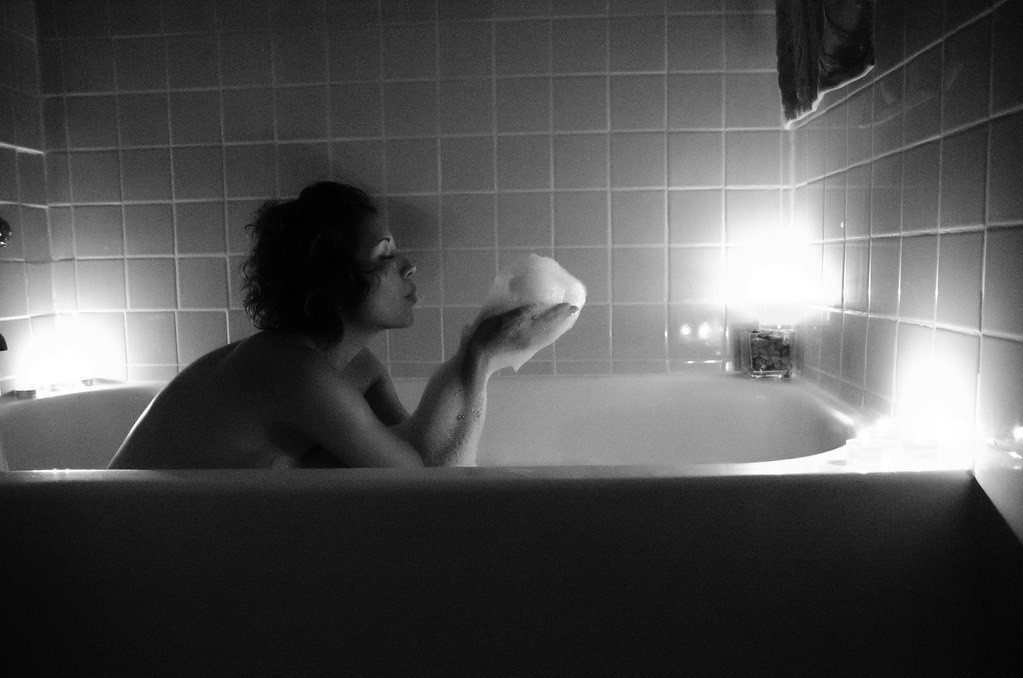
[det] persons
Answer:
[103,183,580,469]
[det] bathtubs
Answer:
[0,361,998,677]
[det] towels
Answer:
[772,1,877,137]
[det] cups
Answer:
[739,329,797,380]
